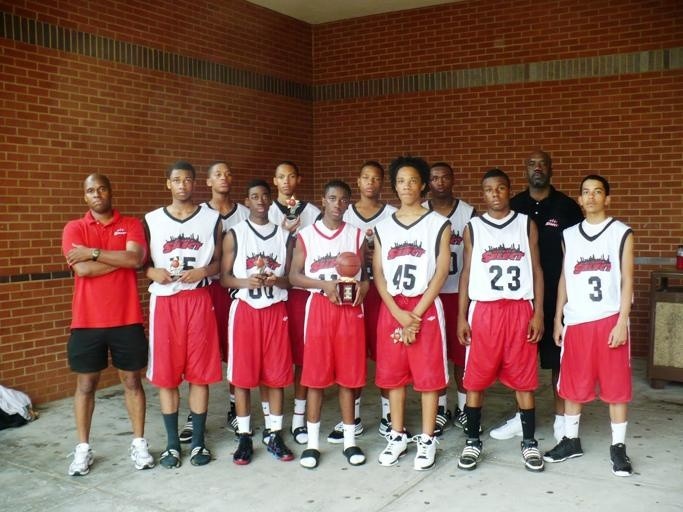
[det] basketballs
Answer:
[333,251,363,278]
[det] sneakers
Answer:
[179,413,192,444]
[377,432,408,467]
[411,433,440,471]
[233,432,254,465]
[542,435,584,463]
[267,431,295,462]
[226,401,238,431]
[609,442,633,477]
[66,442,96,476]
[520,439,545,473]
[377,413,414,444]
[457,439,483,471]
[327,417,365,444]
[433,406,451,437]
[553,415,565,440]
[489,412,523,440]
[453,404,484,435]
[127,438,155,470]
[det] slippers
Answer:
[299,449,321,468]
[159,448,182,470]
[290,426,308,445]
[190,446,212,466]
[342,446,366,466]
[262,429,271,446]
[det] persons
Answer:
[178,162,255,443]
[220,180,295,465]
[543,174,634,477]
[258,161,323,447]
[327,161,412,445]
[288,179,370,469]
[419,162,484,437]
[62,174,155,478]
[373,157,450,471]
[141,164,223,470]
[490,149,585,446]
[457,169,546,471]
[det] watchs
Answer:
[93,247,101,261]
